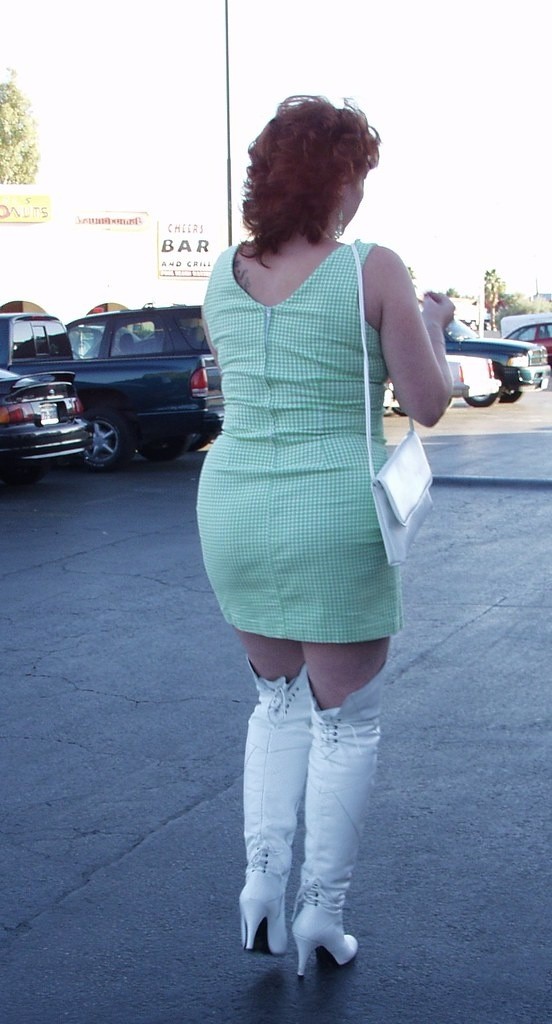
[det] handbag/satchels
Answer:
[370,430,436,568]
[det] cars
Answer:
[501,322,552,368]
[381,351,503,415]
[0,312,87,489]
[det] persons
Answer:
[197,94,456,979]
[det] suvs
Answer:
[60,306,226,476]
[442,318,551,411]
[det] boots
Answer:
[294,656,389,978]
[240,653,310,955]
